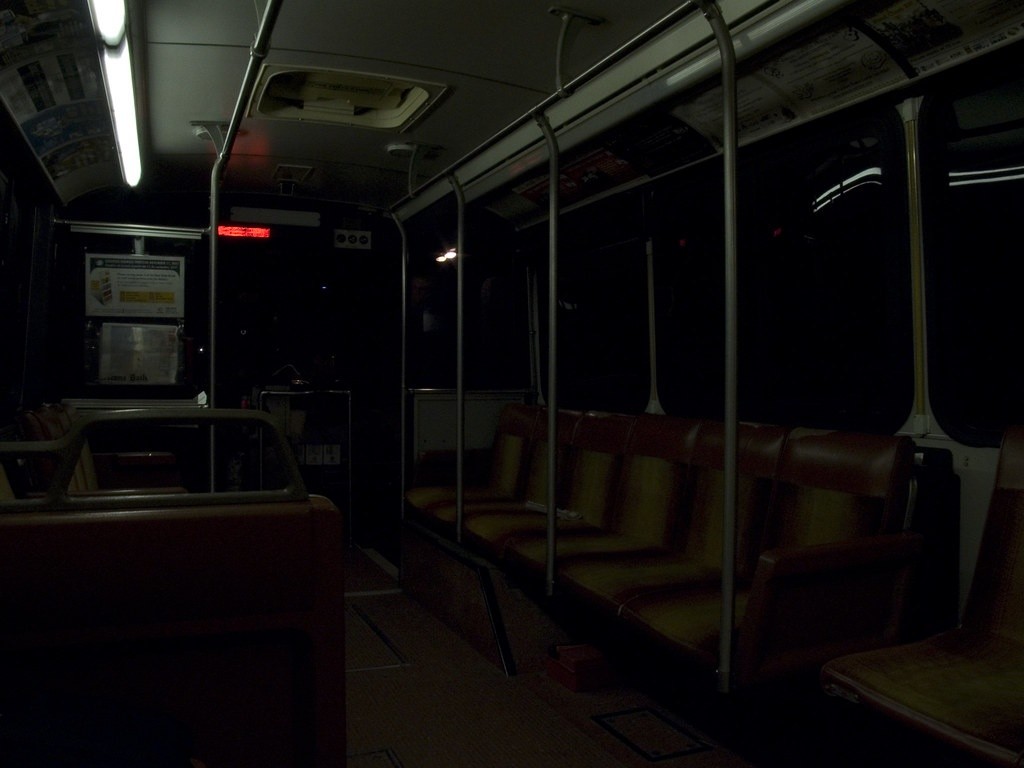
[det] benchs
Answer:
[0,399,350,768]
[406,398,1024,767]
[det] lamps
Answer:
[89,0,143,187]
